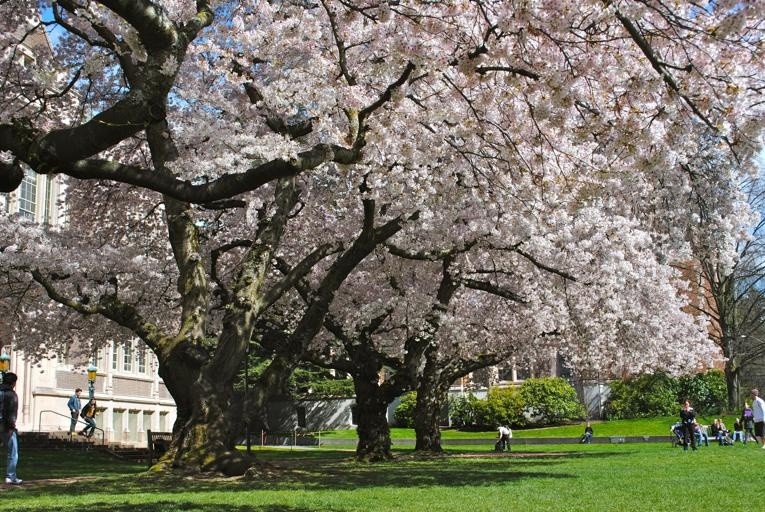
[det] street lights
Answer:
[87,364,97,397]
[0,348,11,377]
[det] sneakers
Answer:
[4,477,22,485]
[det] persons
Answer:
[0,372,22,485]
[503,424,513,446]
[67,388,83,436]
[672,387,765,454]
[79,396,100,439]
[581,423,594,444]
[494,426,511,452]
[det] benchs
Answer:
[147,429,173,466]
[671,425,691,449]
[701,425,730,440]
